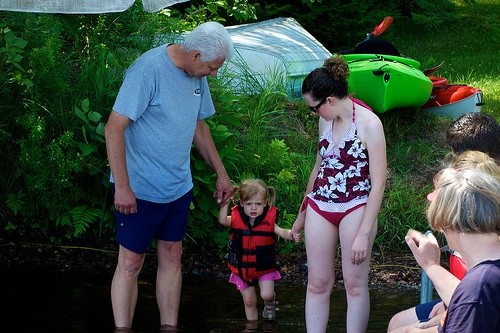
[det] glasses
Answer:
[309,99,326,112]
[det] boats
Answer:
[329,33,435,116]
[415,75,487,125]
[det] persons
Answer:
[387,113,500,333]
[104,22,232,327]
[291,56,386,333]
[219,179,302,319]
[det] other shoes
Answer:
[262,301,276,319]
